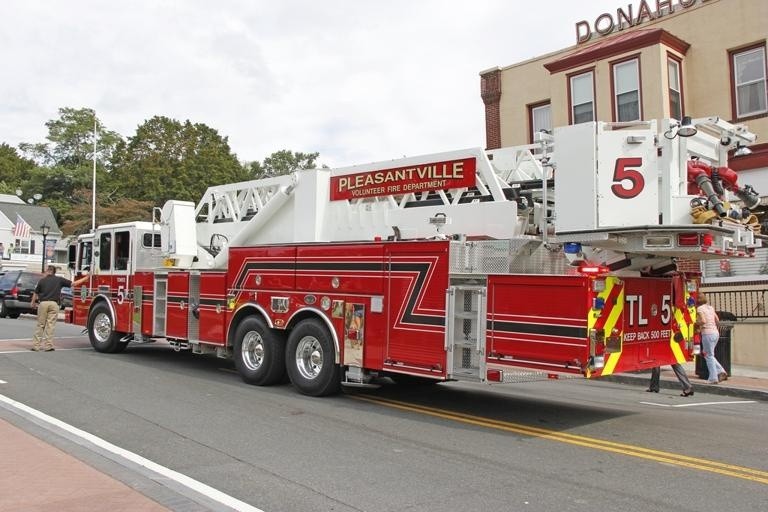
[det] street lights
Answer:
[40,219,50,273]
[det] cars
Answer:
[0,269,74,318]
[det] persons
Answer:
[7,243,16,258]
[643,361,694,396]
[0,242,4,258]
[30,264,93,351]
[696,291,728,384]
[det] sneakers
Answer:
[31,348,36,351]
[45,348,54,351]
[704,372,727,384]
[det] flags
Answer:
[13,214,30,242]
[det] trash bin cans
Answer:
[695,326,734,381]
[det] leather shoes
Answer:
[681,390,694,396]
[646,388,659,393]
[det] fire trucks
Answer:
[57,114,767,399]
